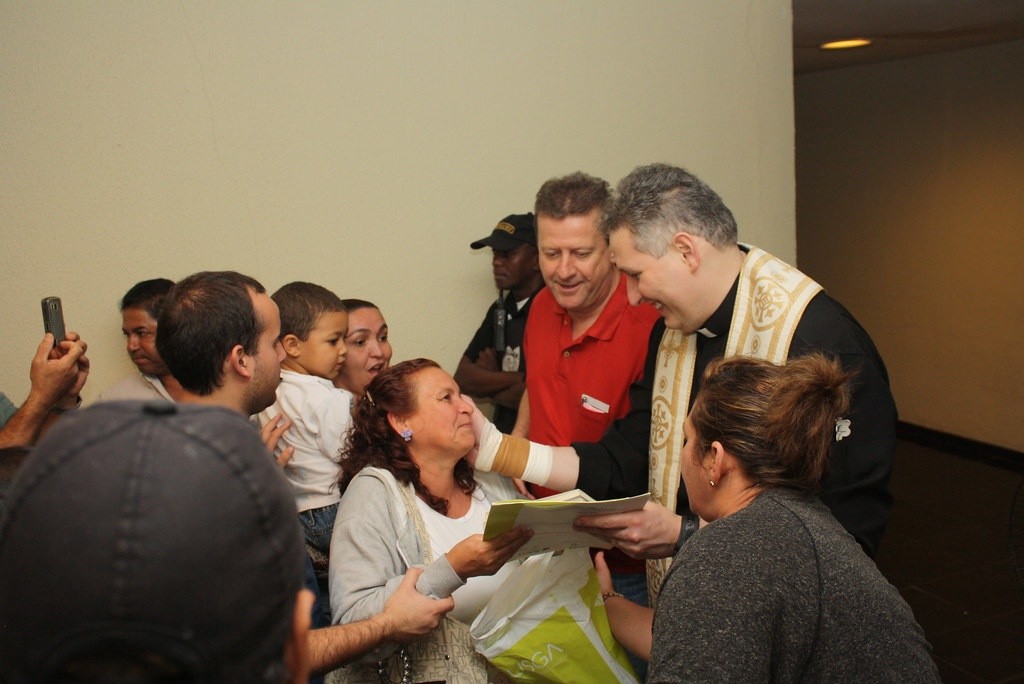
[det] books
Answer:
[480,488,651,560]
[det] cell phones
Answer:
[42,296,66,348]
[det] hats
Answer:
[1,397,304,684]
[470,213,538,250]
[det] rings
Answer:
[615,539,622,549]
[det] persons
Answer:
[242,278,391,684]
[461,162,898,610]
[453,212,546,434]
[328,359,533,684]
[1,398,315,683]
[507,170,663,684]
[156,268,456,675]
[595,353,942,683]
[93,278,184,407]
[0,331,92,520]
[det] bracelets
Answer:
[51,392,83,413]
[674,514,699,555]
[602,590,624,600]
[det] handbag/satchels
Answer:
[323,466,489,684]
[468,547,641,684]
